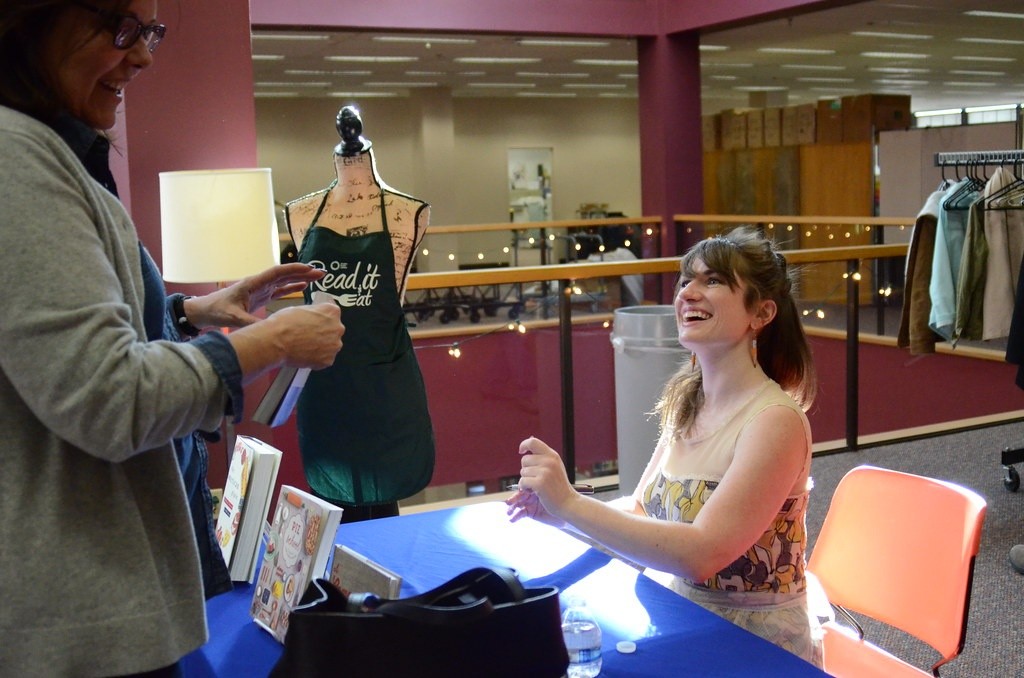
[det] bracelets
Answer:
[174,295,201,335]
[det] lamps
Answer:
[160,167,281,470]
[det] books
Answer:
[214,434,403,646]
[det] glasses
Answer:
[79,0,166,54]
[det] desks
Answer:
[179,500,835,678]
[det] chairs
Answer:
[808,465,987,678]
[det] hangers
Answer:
[942,157,1024,211]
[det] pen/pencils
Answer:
[506,484,595,493]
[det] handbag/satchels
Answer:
[270,566,571,678]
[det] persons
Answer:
[507,229,824,672]
[0,0,346,678]
[280,145,433,503]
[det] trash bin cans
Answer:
[611,305,693,498]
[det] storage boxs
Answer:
[698,92,912,149]
